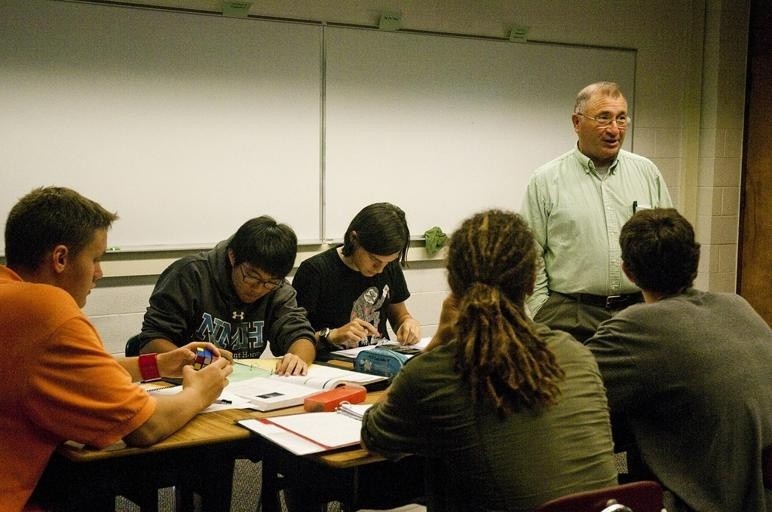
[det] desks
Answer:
[57,359,392,512]
[260,388,422,512]
[325,334,437,377]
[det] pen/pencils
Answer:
[631,199,637,214]
[215,397,232,406]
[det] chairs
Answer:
[535,480,663,512]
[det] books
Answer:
[138,336,432,456]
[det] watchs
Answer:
[319,327,332,347]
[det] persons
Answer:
[359,208,619,512]
[291,202,421,362]
[519,81,674,344]
[0,185,233,512]
[138,216,316,376]
[584,207,772,512]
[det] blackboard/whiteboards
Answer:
[1,0,637,257]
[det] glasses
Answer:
[576,113,631,130]
[239,266,287,292]
